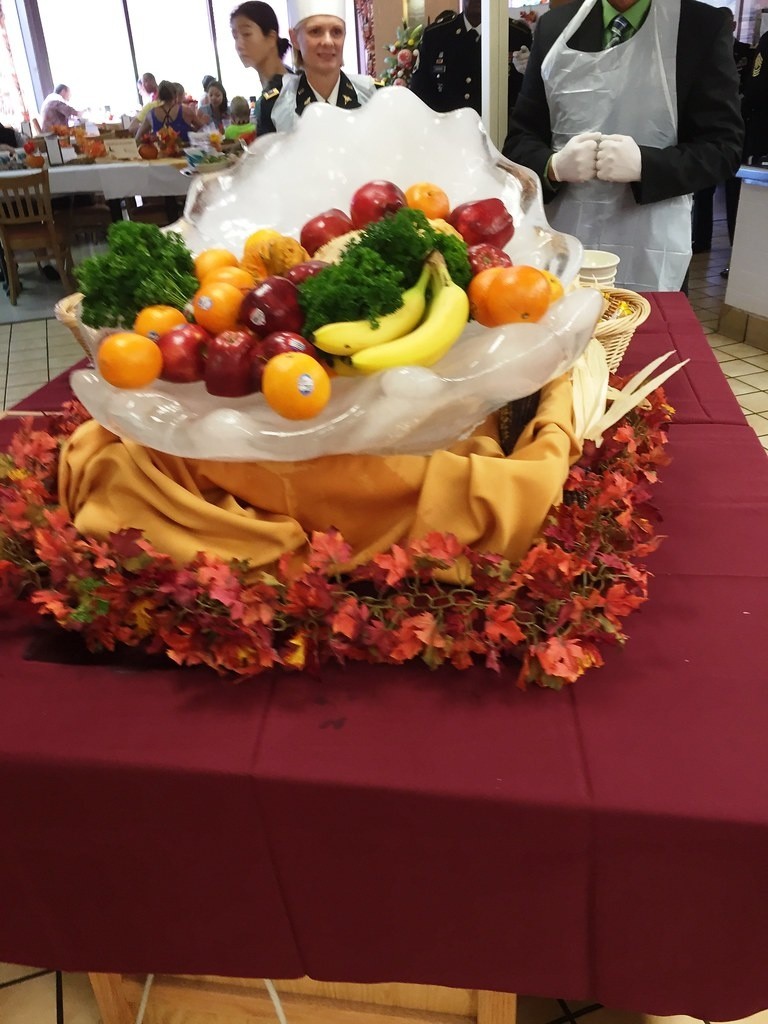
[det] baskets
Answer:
[55,290,650,373]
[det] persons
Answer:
[40,84,87,136]
[411,0,533,118]
[126,73,257,145]
[501,0,745,293]
[230,1,295,139]
[260,0,378,138]
[716,7,768,168]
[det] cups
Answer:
[579,249,620,290]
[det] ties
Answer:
[467,29,480,55]
[605,16,629,49]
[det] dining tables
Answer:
[0,160,137,298]
[0,123,219,171]
[98,147,193,225]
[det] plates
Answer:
[179,167,196,178]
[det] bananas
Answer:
[309,249,470,377]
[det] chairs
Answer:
[20,116,211,245]
[0,168,76,306]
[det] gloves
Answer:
[550,132,601,183]
[596,134,641,183]
[513,46,530,74]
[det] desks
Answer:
[717,164,768,351]
[1,290,768,1022]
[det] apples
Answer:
[153,180,513,397]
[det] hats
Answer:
[286,0,345,31]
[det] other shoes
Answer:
[5,282,23,296]
[692,239,712,252]
[39,262,60,283]
[721,269,730,279]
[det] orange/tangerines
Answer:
[97,231,331,421]
[467,263,561,327]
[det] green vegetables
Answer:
[70,220,201,331]
